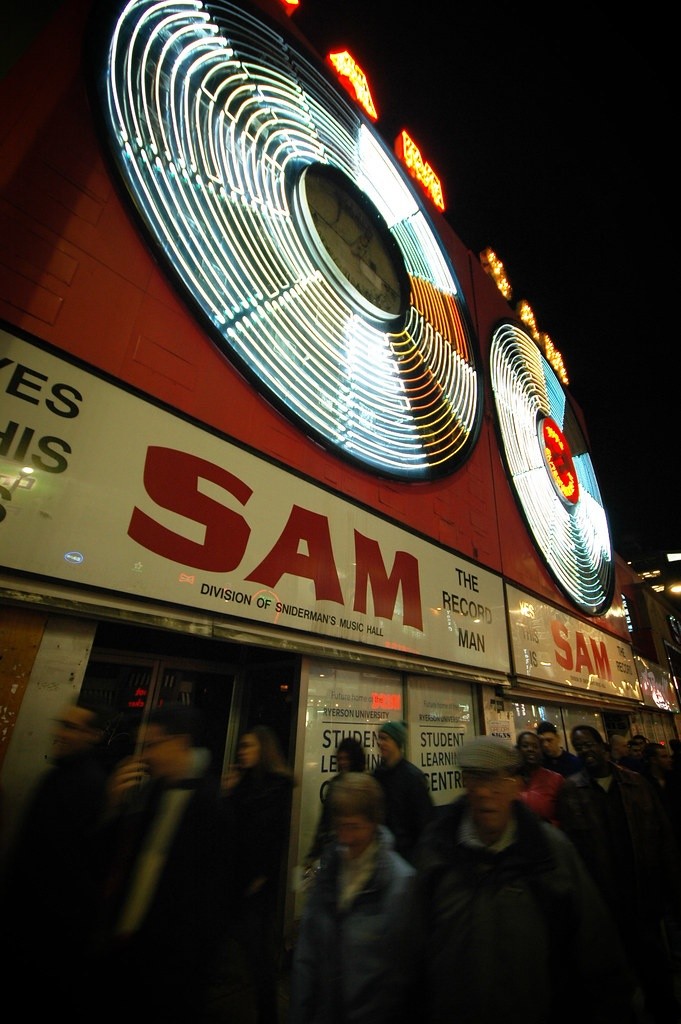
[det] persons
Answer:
[1,702,681,1024]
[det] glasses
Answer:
[461,771,518,791]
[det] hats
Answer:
[459,740,531,777]
[382,721,410,746]
